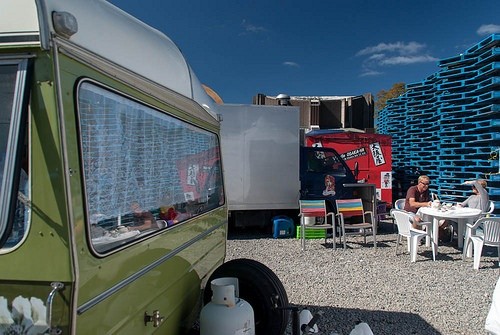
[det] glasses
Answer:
[420,181,430,187]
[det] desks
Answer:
[417,205,482,255]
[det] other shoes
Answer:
[439,226,444,236]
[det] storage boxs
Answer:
[297,225,327,239]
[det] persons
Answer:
[451,179,489,237]
[157,197,183,226]
[403,175,449,246]
[89,211,108,240]
[125,201,158,231]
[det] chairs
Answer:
[299,200,336,251]
[390,199,500,263]
[336,199,377,249]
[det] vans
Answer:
[0,0,228,335]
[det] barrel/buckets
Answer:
[272,215,295,238]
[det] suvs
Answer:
[299,146,359,200]
